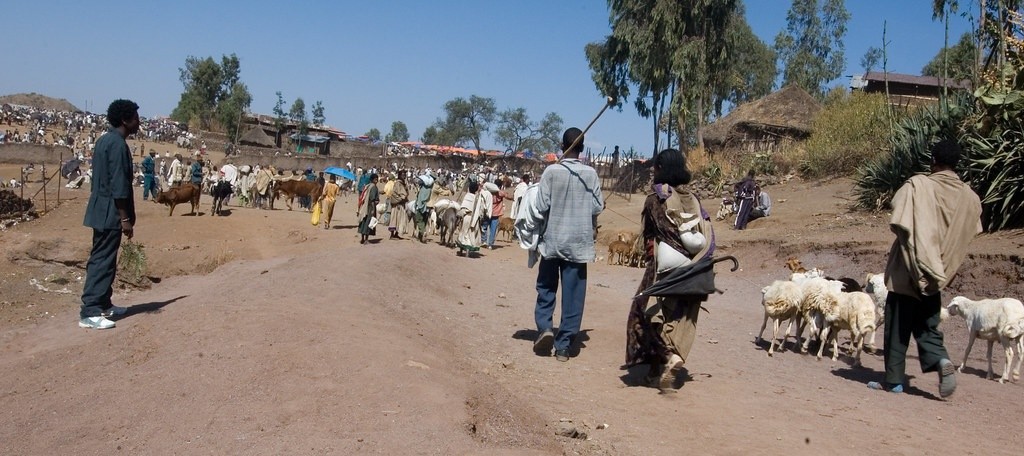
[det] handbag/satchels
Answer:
[367,215,378,230]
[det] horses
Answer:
[209,179,235,216]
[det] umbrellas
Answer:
[632,255,739,299]
[325,166,357,181]
[62,160,83,177]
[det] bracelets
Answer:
[120,218,130,222]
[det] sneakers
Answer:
[101,305,127,318]
[79,314,116,329]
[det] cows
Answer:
[157,183,201,217]
[272,179,323,213]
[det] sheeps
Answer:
[607,231,646,268]
[753,266,948,367]
[495,217,518,243]
[946,295,1024,384]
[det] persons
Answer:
[142,148,531,257]
[620,149,716,389]
[10,177,16,188]
[78,99,140,329]
[26,163,34,170]
[514,128,604,361]
[733,169,771,230]
[0,103,206,163]
[612,146,619,159]
[867,140,983,398]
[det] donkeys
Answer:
[434,206,460,248]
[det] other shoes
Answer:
[480,242,487,247]
[466,250,470,256]
[488,246,493,250]
[456,251,462,256]
[360,240,373,245]
[555,350,569,361]
[533,329,554,355]
[938,358,957,397]
[867,381,903,393]
[390,235,404,240]
[659,353,683,386]
